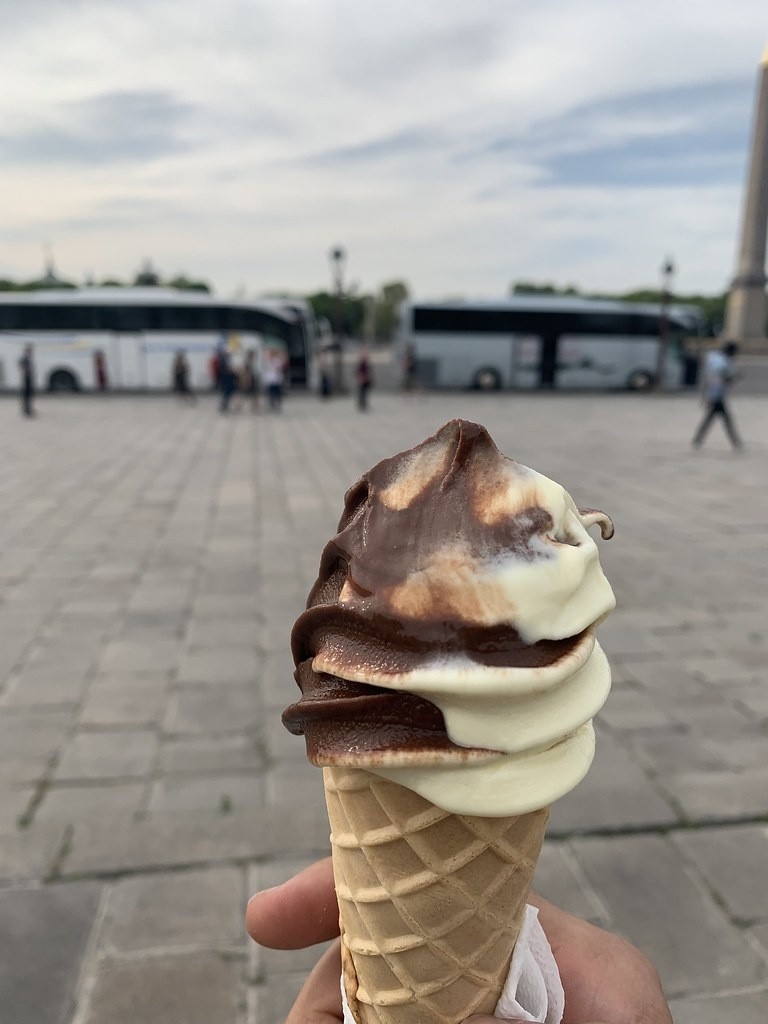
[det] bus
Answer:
[0,289,319,395]
[398,295,704,393]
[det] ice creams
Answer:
[283,418,615,1024]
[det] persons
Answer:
[691,341,742,448]
[216,350,285,410]
[354,353,372,411]
[245,857,672,1024]
[173,350,191,404]
[18,343,35,416]
